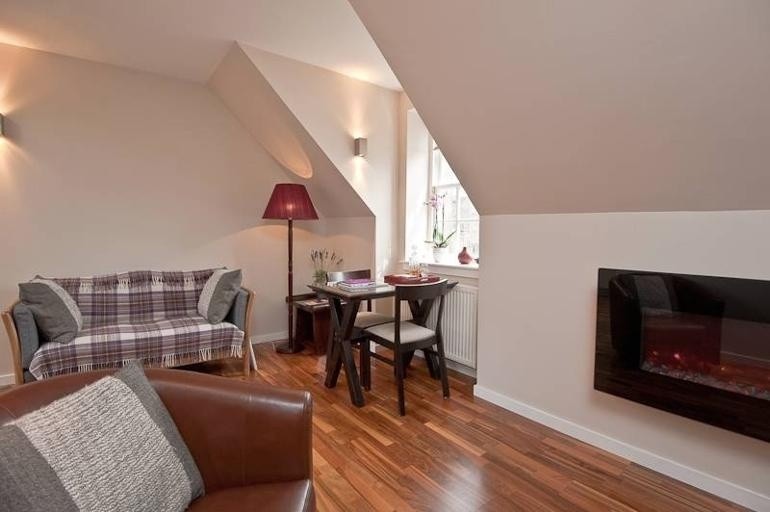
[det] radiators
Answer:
[392,282,479,369]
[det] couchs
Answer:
[0,267,257,385]
[0,367,316,512]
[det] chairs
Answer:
[364,279,450,416]
[325,269,396,387]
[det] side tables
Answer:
[286,293,347,356]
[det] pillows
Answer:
[197,268,243,324]
[18,274,83,345]
[1,360,206,512]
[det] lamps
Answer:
[262,183,319,354]
[354,138,368,157]
[0,113,6,138]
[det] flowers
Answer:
[422,187,457,247]
[310,248,344,284]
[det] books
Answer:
[338,278,375,291]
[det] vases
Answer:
[312,275,326,289]
[432,247,448,264]
